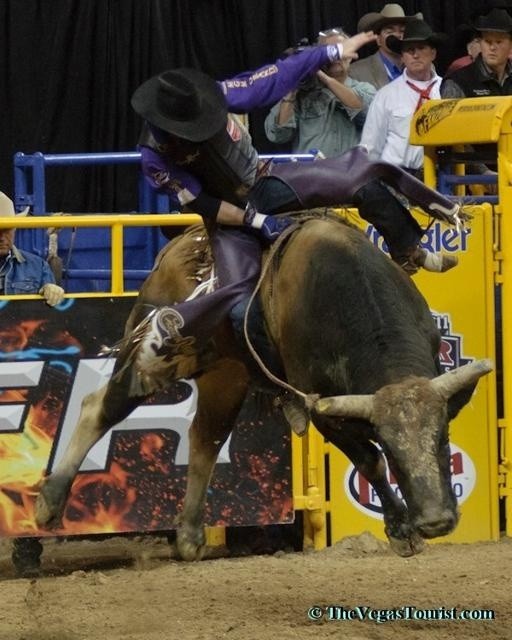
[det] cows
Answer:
[35,208,493,562]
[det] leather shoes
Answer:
[404,248,456,273]
[274,391,309,436]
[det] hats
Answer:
[0,191,30,217]
[358,4,512,53]
[131,67,226,142]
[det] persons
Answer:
[0,191,66,307]
[133,30,459,436]
[346,3,432,93]
[440,8,512,196]
[356,18,444,206]
[262,25,379,161]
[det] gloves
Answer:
[243,200,291,240]
[39,284,65,307]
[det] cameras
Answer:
[283,37,328,92]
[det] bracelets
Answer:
[279,96,297,103]
